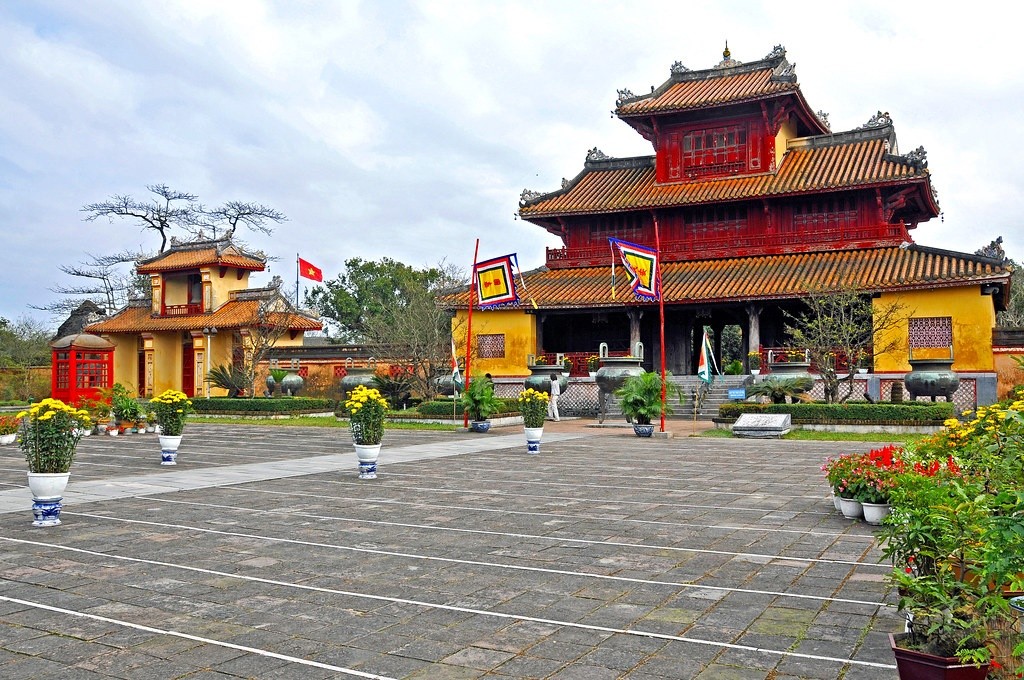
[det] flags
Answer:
[452,341,461,384]
[477,255,539,310]
[607,238,660,302]
[299,258,322,282]
[698,334,724,382]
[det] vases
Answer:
[857,368,869,374]
[0,433,17,445]
[524,426,544,455]
[589,372,597,377]
[830,490,841,510]
[158,435,183,466]
[27,471,71,526]
[751,370,760,375]
[861,503,888,525]
[889,507,912,527]
[840,497,863,519]
[352,442,382,480]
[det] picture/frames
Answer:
[561,372,570,377]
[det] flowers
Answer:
[747,350,762,370]
[343,385,389,445]
[559,356,573,373]
[585,355,600,372]
[0,415,21,435]
[149,388,193,436]
[517,388,550,428]
[535,356,548,364]
[820,389,1024,502]
[16,397,90,473]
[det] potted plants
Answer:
[872,502,1024,680]
[69,383,160,436]
[611,369,686,437]
[460,377,505,432]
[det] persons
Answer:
[547,373,560,422]
[485,374,494,391]
